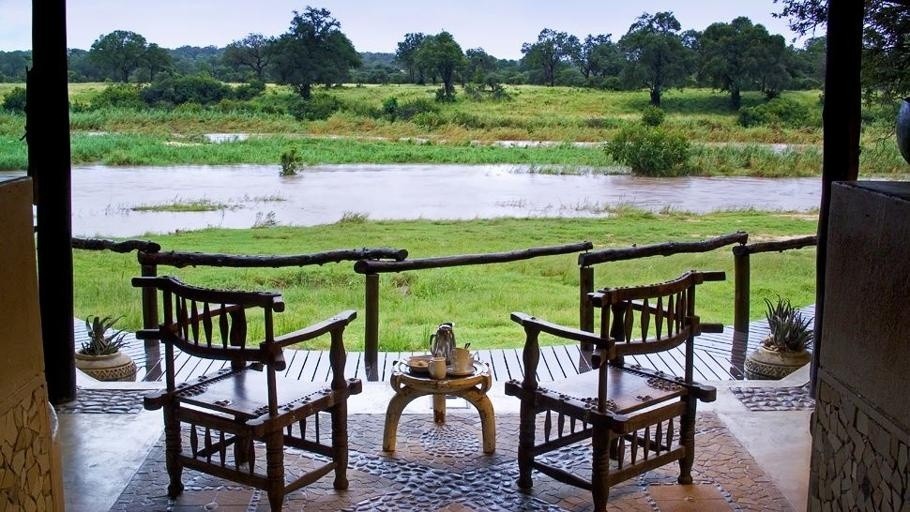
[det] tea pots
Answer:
[429,323,455,366]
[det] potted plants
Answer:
[744,294,814,380]
[74,315,136,381]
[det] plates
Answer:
[447,367,475,376]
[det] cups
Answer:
[428,357,446,379]
[452,347,469,372]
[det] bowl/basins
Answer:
[407,358,430,372]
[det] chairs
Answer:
[505,269,725,512]
[131,276,362,512]
